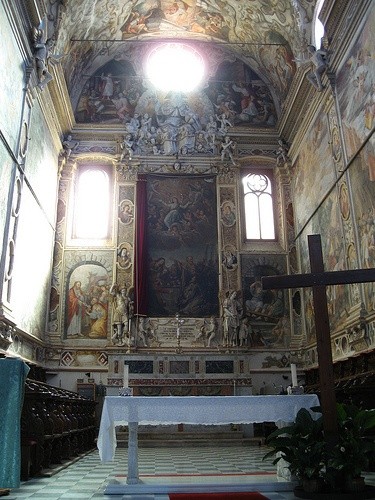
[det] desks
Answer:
[97,394,327,489]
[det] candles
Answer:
[291,364,298,386]
[123,365,128,387]
[177,328,180,336]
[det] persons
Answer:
[107,281,149,347]
[197,291,249,348]
[291,44,328,92]
[62,135,80,161]
[33,31,53,92]
[276,139,291,166]
[118,84,238,169]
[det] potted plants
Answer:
[259,402,375,493]
